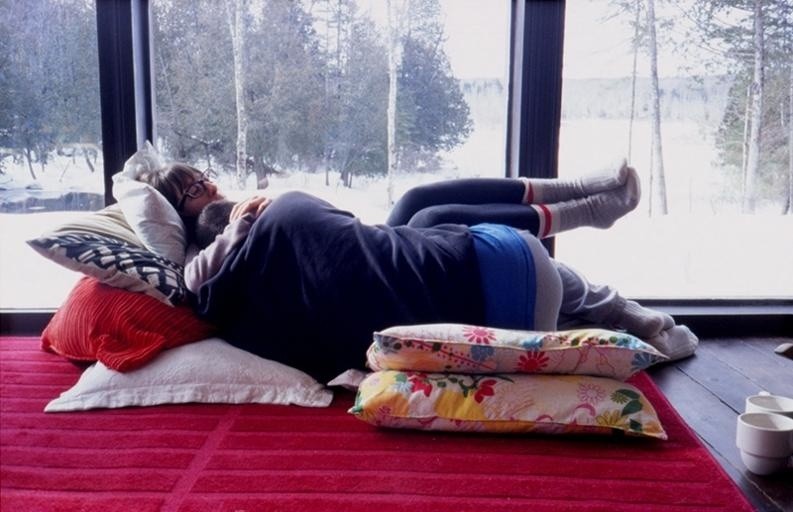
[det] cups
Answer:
[736,391,793,475]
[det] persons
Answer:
[144,157,643,298]
[194,190,700,387]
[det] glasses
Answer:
[178,168,222,209]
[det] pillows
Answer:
[38,273,205,374]
[26,202,194,310]
[326,368,367,389]
[347,371,668,441]
[112,141,186,262]
[365,324,669,382]
[43,336,334,413]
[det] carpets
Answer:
[1,334,757,512]
